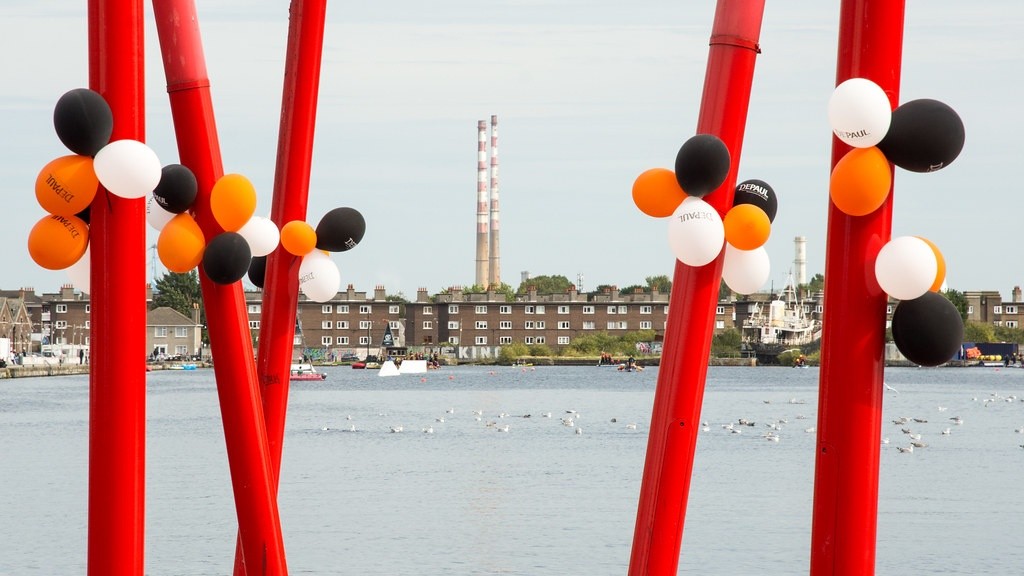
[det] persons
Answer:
[0,349,26,368]
[150,347,159,361]
[298,356,303,375]
[79,349,89,364]
[394,351,438,369]
[602,354,636,366]
[1003,352,1024,366]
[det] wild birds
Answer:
[884,382,899,394]
[470,409,551,433]
[702,419,756,434]
[881,416,962,454]
[971,393,1024,408]
[762,398,807,405]
[763,414,817,441]
[560,409,638,436]
[939,406,948,413]
[320,406,453,435]
[1015,426,1024,449]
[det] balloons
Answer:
[632,136,732,267]
[248,254,268,288]
[874,234,963,366]
[54,88,112,157]
[721,178,778,296]
[157,210,256,284]
[210,174,280,258]
[92,138,162,199]
[281,207,366,303]
[828,77,964,215]
[28,154,100,271]
[145,164,198,230]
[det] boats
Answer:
[289,364,326,381]
[169,365,196,370]
[353,362,381,369]
[597,362,642,371]
[742,268,815,345]
[794,361,811,368]
